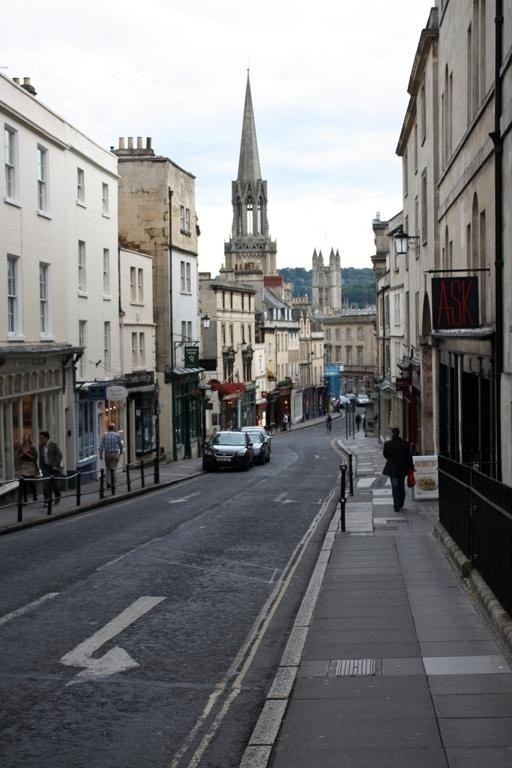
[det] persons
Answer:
[16,431,63,507]
[324,414,332,430]
[356,409,367,431]
[282,412,288,431]
[382,427,415,513]
[98,422,123,489]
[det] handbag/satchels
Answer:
[407,469,416,488]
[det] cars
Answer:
[331,392,370,409]
[202,426,271,474]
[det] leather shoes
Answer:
[44,499,60,507]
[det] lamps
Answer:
[391,227,419,261]
[201,311,212,329]
